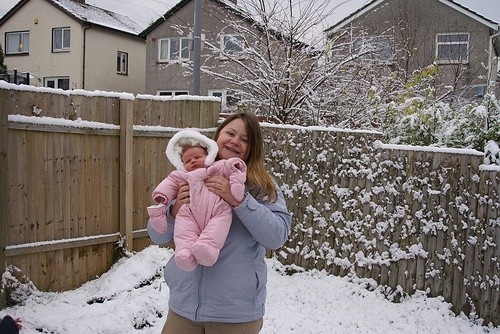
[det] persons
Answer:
[152,129,247,272]
[147,112,291,334]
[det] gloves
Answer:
[147,204,169,234]
[229,173,247,201]
